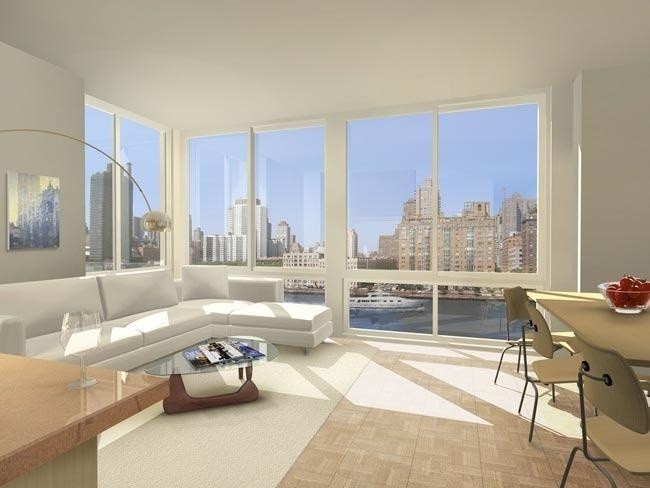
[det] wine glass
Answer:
[58,311,106,391]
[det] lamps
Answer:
[1,129,174,233]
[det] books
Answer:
[181,339,266,370]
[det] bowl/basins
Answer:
[597,281,650,314]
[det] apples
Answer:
[605,277,650,308]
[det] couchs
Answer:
[1,265,334,372]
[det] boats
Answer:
[350,289,423,309]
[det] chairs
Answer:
[494,286,650,488]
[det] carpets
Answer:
[97,336,382,488]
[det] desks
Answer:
[0,353,170,488]
